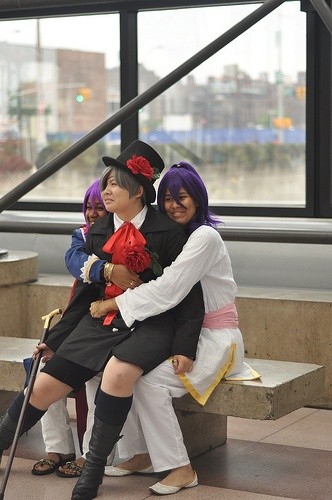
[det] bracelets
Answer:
[92,300,102,316]
[103,262,116,288]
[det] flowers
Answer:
[126,154,160,182]
[122,242,163,278]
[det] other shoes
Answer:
[103,465,155,476]
[149,471,198,495]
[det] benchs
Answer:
[1,336,325,460]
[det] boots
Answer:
[71,415,123,500]
[0,408,28,465]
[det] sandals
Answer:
[55,462,83,477]
[31,452,76,475]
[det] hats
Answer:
[101,140,164,204]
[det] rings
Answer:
[171,360,177,364]
[130,281,134,286]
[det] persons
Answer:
[0,141,205,500]
[26,179,143,479]
[87,161,262,494]
